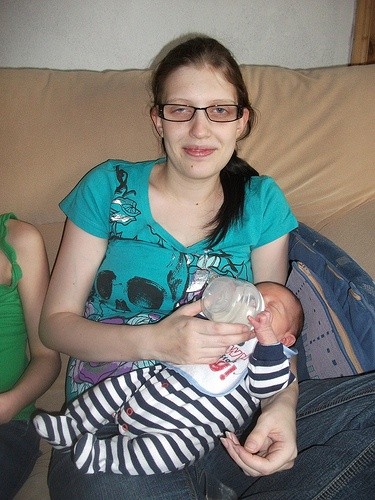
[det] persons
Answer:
[40,37,375,500]
[34,282,305,475]
[0,211,61,500]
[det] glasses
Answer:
[157,103,244,123]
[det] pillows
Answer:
[288,221,375,371]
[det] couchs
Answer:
[0,63,375,500]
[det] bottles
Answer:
[201,276,265,331]
